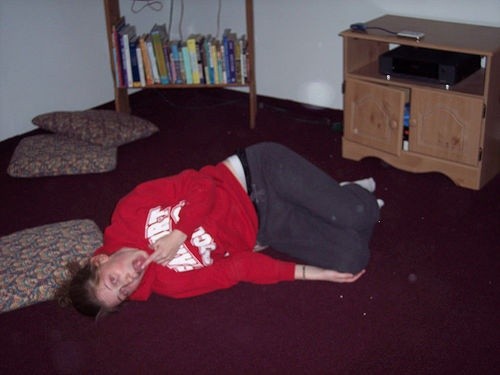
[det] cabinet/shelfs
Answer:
[338,13,500,192]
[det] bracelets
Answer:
[302,264,305,281]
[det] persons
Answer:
[53,141,387,324]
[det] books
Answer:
[112,16,250,89]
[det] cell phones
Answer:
[397,30,424,40]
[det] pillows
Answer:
[29,110,160,147]
[7,133,116,177]
[0,219,103,314]
